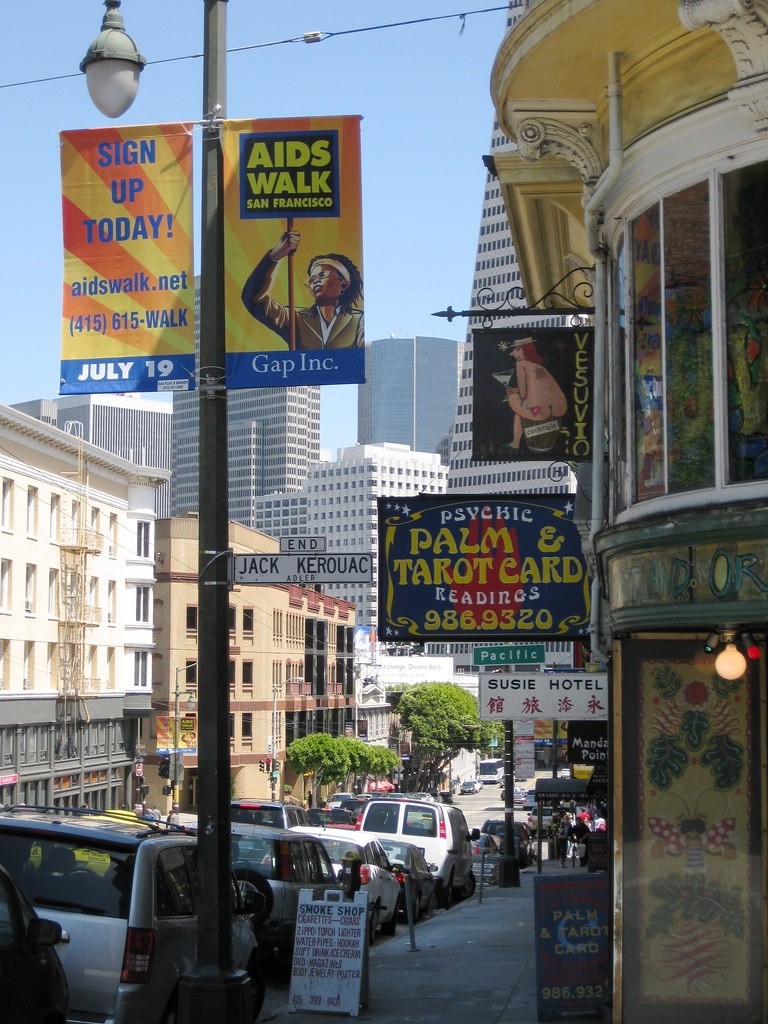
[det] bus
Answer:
[478,757,504,784]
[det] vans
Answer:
[356,796,480,910]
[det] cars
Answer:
[460,780,484,795]
[467,828,498,859]
[501,786,537,810]
[0,864,71,1024]
[306,791,454,831]
[259,826,403,948]
[374,836,438,926]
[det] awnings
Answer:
[535,778,589,874]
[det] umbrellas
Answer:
[370,780,395,791]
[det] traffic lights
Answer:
[158,755,170,779]
[272,759,280,772]
[259,760,264,771]
[266,759,270,772]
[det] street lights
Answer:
[353,695,386,796]
[175,689,197,813]
[272,676,304,802]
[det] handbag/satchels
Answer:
[556,823,564,835]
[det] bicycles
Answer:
[571,839,578,868]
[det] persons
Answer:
[166,810,179,829]
[133,803,161,828]
[307,791,312,808]
[80,804,89,816]
[560,808,606,867]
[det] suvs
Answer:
[526,806,560,837]
[479,818,537,870]
[0,803,266,1024]
[230,796,320,861]
[158,809,345,984]
